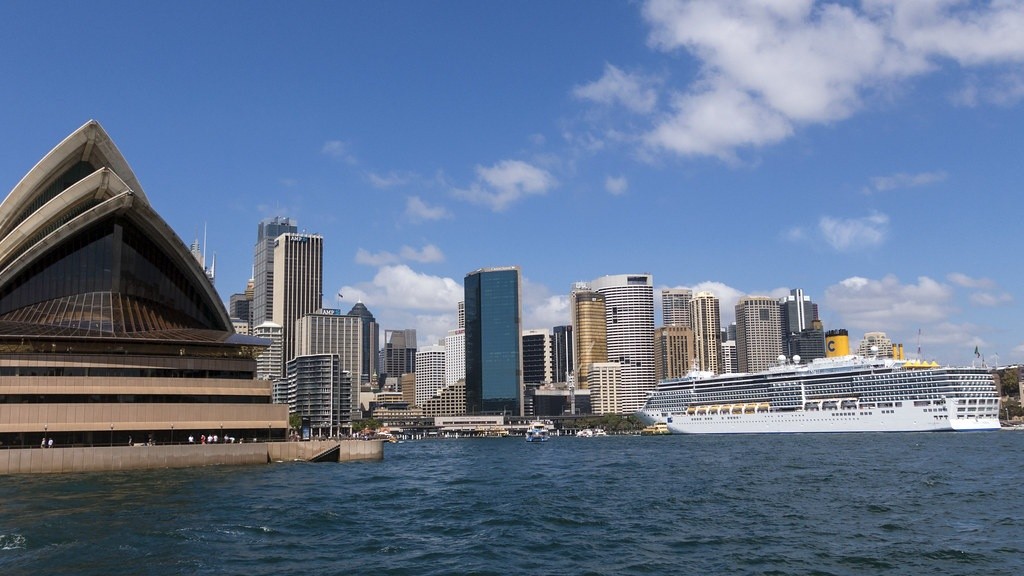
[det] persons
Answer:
[213,434,218,444]
[145,432,153,446]
[223,433,246,443]
[48,438,53,448]
[188,434,194,445]
[41,438,45,448]
[207,434,213,443]
[200,434,206,444]
[127,434,134,446]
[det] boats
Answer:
[576,428,611,437]
[640,423,672,436]
[524,415,551,442]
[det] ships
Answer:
[633,349,1005,432]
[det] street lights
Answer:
[294,421,351,438]
[44,424,48,447]
[268,421,272,442]
[170,423,174,445]
[220,423,224,444]
[110,423,114,447]
